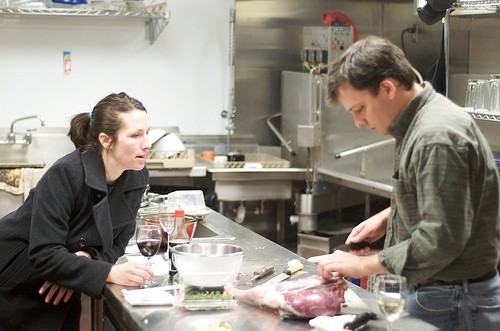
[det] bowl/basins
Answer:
[141,213,198,243]
[169,242,248,288]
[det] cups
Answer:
[464,73,500,115]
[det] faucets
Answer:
[7,115,44,143]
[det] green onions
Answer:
[186,291,234,300]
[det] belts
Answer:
[422,268,498,287]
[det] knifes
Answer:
[247,265,274,283]
[332,241,371,253]
[260,263,304,285]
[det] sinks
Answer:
[198,152,292,201]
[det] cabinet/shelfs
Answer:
[444,3,500,123]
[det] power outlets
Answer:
[410,24,418,43]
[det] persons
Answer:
[0,92,154,331]
[318,34,499,331]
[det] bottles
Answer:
[167,208,190,278]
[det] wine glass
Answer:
[374,274,407,331]
[158,202,180,262]
[135,224,163,289]
